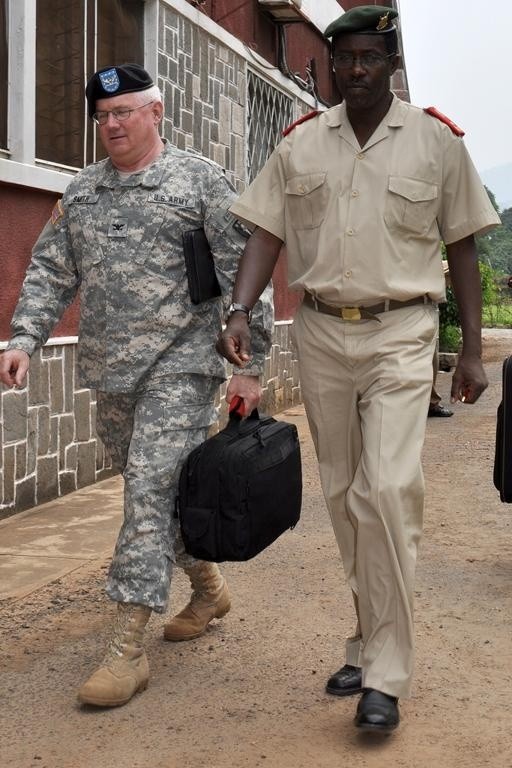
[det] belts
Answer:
[303,289,432,323]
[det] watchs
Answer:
[223,302,252,324]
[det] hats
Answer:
[323,5,398,39]
[85,63,155,120]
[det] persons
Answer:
[0,64,273,708]
[215,4,502,733]
[427,330,454,417]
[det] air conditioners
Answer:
[258,0,312,25]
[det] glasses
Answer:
[330,47,399,70]
[91,100,154,126]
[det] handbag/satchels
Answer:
[181,227,222,306]
[173,396,302,563]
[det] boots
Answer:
[163,559,232,641]
[78,601,152,707]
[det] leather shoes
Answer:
[427,405,454,417]
[325,664,366,697]
[354,689,399,729]
